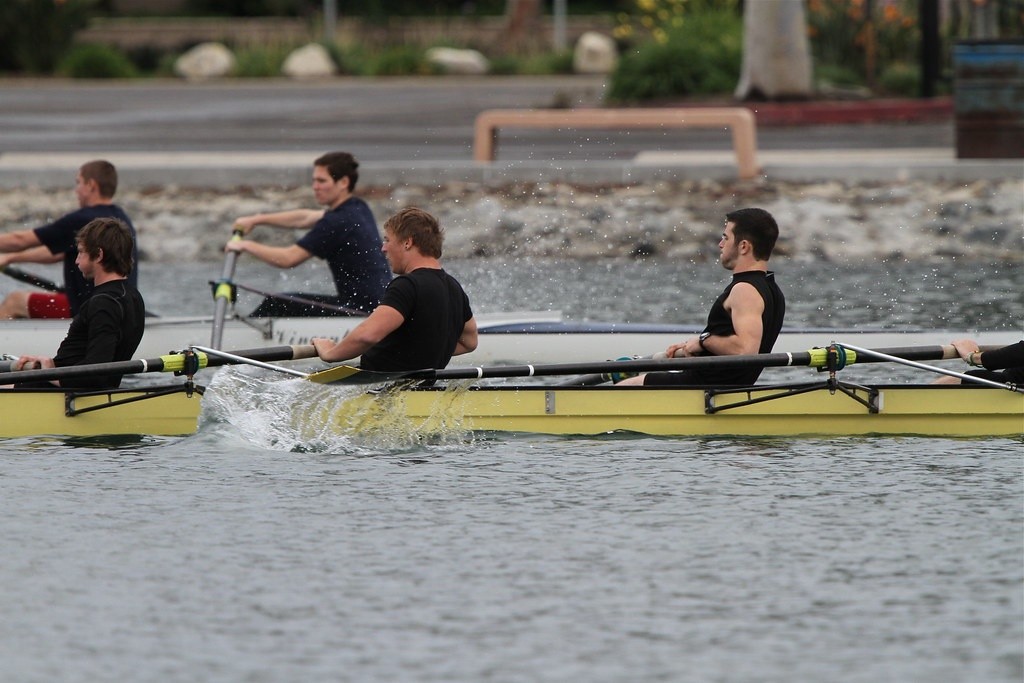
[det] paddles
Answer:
[0,264,159,317]
[307,344,1009,384]
[211,225,241,352]
[0,344,318,384]
[552,346,684,387]
[0,360,40,373]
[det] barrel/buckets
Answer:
[949,38,1024,159]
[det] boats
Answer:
[0,276,1024,445]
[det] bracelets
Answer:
[966,350,983,368]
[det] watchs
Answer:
[699,332,711,351]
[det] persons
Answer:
[935,340,1024,384]
[224,151,392,317]
[312,207,478,386]
[614,207,785,385]
[0,160,138,319]
[0,217,145,388]
